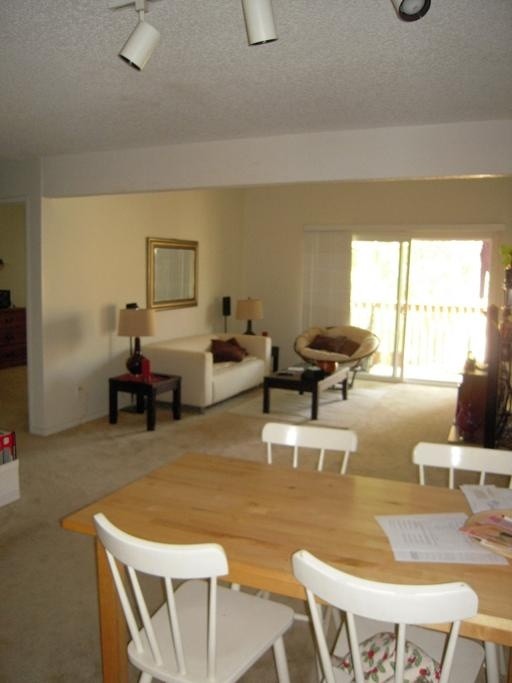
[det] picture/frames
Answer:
[147,238,199,311]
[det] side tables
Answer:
[109,372,182,431]
[271,346,279,372]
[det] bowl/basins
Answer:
[318,360,339,371]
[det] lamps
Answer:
[241,0,279,46]
[117,309,154,375]
[237,296,263,335]
[391,0,431,21]
[119,0,159,71]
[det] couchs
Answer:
[142,334,272,414]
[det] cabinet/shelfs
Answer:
[0,307,27,369]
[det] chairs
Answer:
[231,423,357,621]
[292,549,485,683]
[412,442,512,676]
[294,324,379,390]
[92,513,293,683]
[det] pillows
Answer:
[212,339,245,362]
[306,334,347,352]
[226,338,248,356]
[340,340,361,357]
